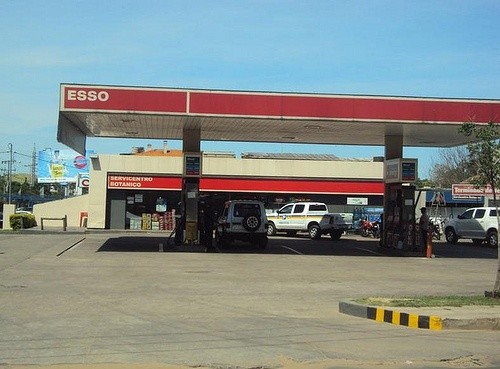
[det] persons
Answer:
[419,207,429,249]
[205,205,215,248]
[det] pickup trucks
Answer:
[265,201,354,241]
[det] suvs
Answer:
[214,199,269,252]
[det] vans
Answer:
[442,206,500,248]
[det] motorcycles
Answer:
[358,219,382,239]
